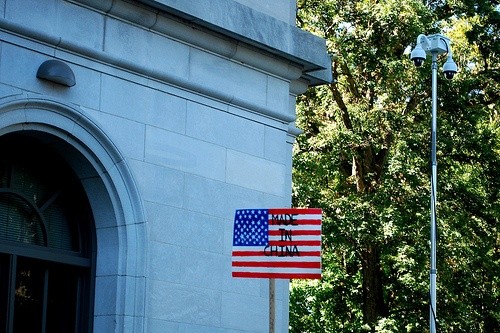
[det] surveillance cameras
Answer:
[411,45,426,67]
[443,59,458,79]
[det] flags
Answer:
[232,209,322,279]
